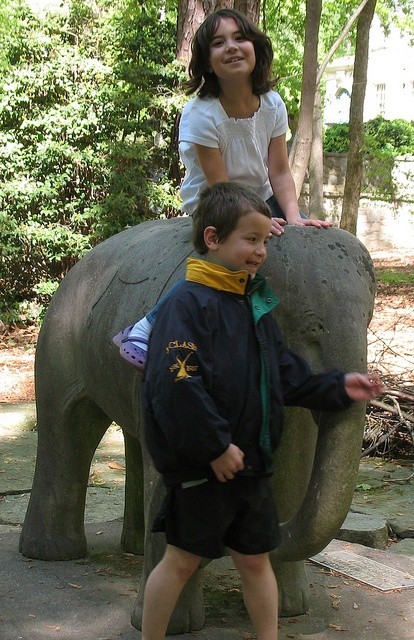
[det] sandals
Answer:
[112,325,149,370]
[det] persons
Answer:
[113,9,335,371]
[140,183,384,639]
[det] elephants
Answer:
[18,215,378,634]
